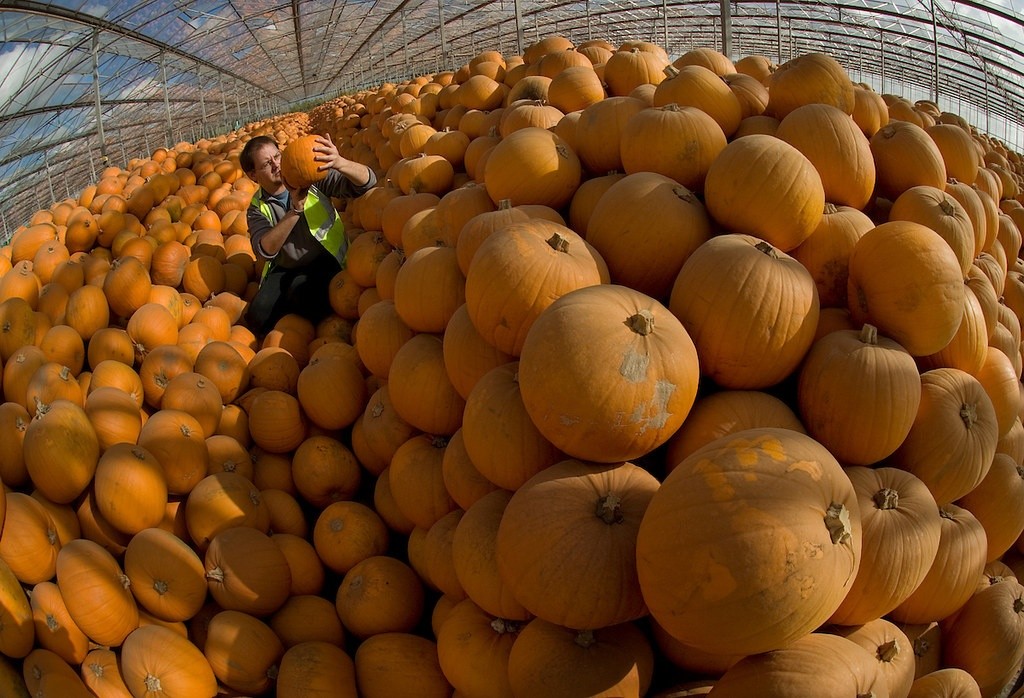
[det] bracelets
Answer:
[290,199,304,216]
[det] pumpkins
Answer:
[0,34,1024,698]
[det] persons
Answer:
[237,132,381,341]
